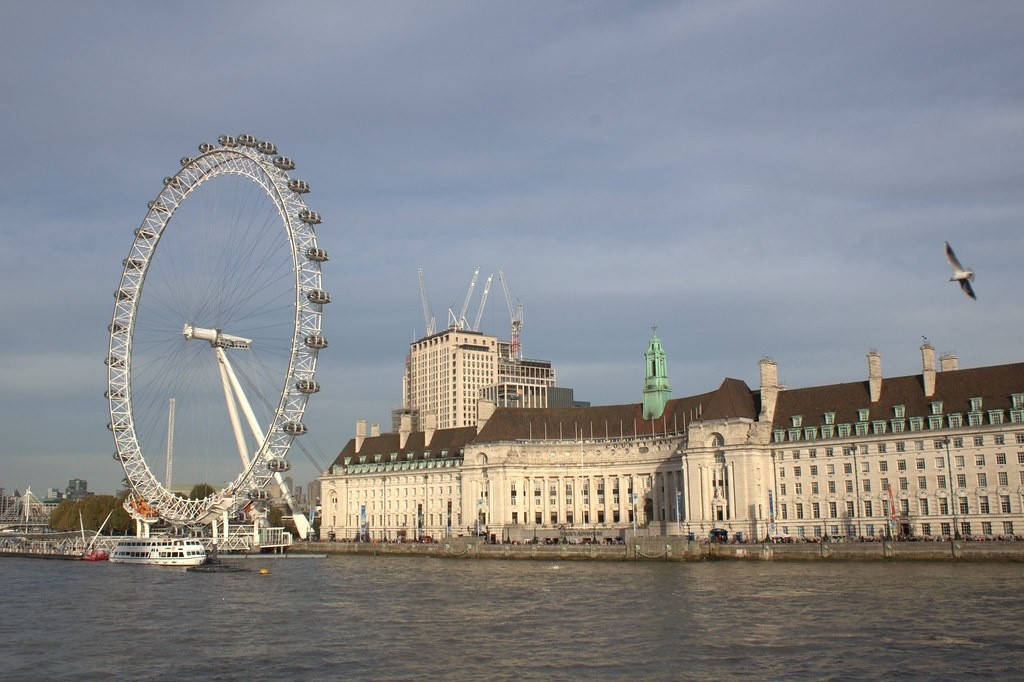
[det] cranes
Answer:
[415,260,527,362]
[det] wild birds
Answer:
[944,240,977,302]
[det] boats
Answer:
[107,536,207,566]
